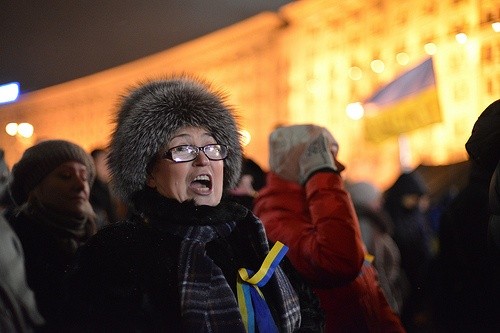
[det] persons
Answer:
[0,71,500,333]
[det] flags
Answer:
[357,56,444,146]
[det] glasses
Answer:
[154,143,229,163]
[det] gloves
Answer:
[298,134,339,185]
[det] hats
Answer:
[12,139,95,207]
[269,125,345,182]
[103,72,245,208]
